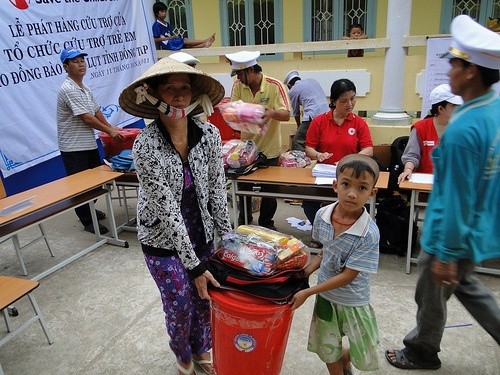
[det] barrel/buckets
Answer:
[207,97,241,142]
[98,127,142,157]
[208,288,294,375]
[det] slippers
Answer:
[385,349,442,370]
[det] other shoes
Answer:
[95,209,105,219]
[84,222,108,234]
[267,225,276,230]
[231,216,253,229]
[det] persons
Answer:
[341,23,369,57]
[231,51,291,232]
[385,15,500,370]
[288,154,381,375]
[302,79,373,255]
[398,83,464,184]
[118,57,234,375]
[284,70,329,151]
[169,52,200,68]
[56,47,126,235]
[152,2,216,51]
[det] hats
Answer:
[429,84,463,105]
[440,15,500,69]
[283,71,302,86]
[118,56,225,119]
[168,52,200,63]
[60,46,88,63]
[225,51,260,78]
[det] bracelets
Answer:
[316,152,319,159]
[107,128,112,134]
[405,167,413,172]
[108,123,113,128]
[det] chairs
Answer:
[0,275,53,375]
[389,137,424,223]
[0,177,55,275]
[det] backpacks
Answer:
[376,195,419,254]
[110,150,136,171]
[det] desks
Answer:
[227,166,390,253]
[93,165,140,237]
[399,177,500,274]
[0,169,129,282]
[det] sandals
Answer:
[175,357,197,375]
[192,351,217,375]
